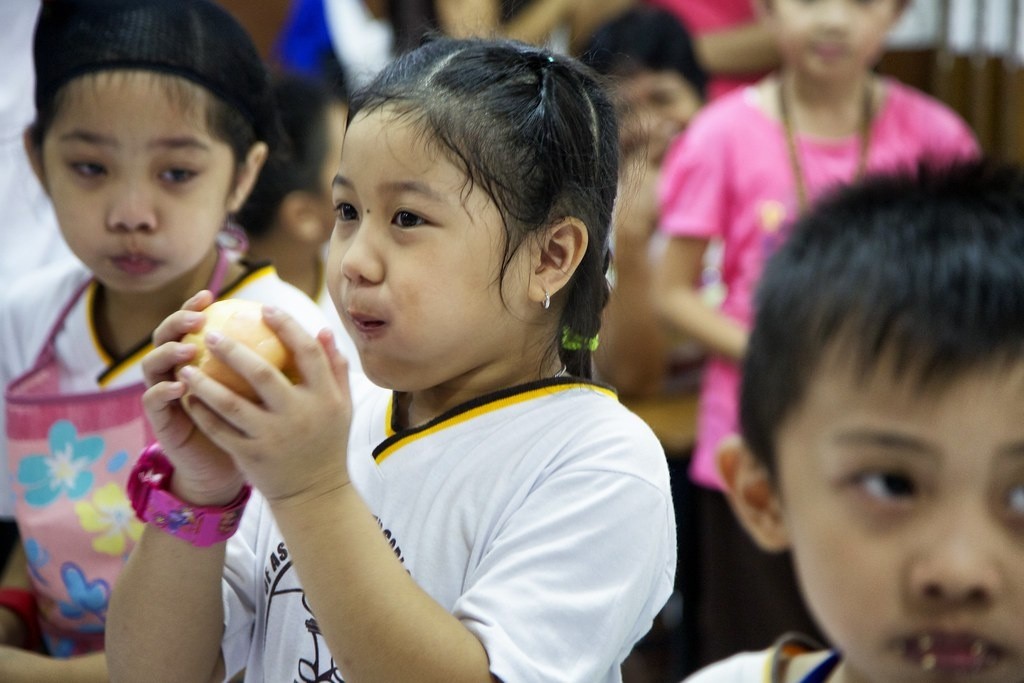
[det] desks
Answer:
[619,387,742,683]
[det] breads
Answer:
[174,297,299,406]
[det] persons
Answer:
[0,0,331,682]
[104,35,678,683]
[680,155,1024,682]
[268,1,988,683]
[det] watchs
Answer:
[124,439,253,550]
